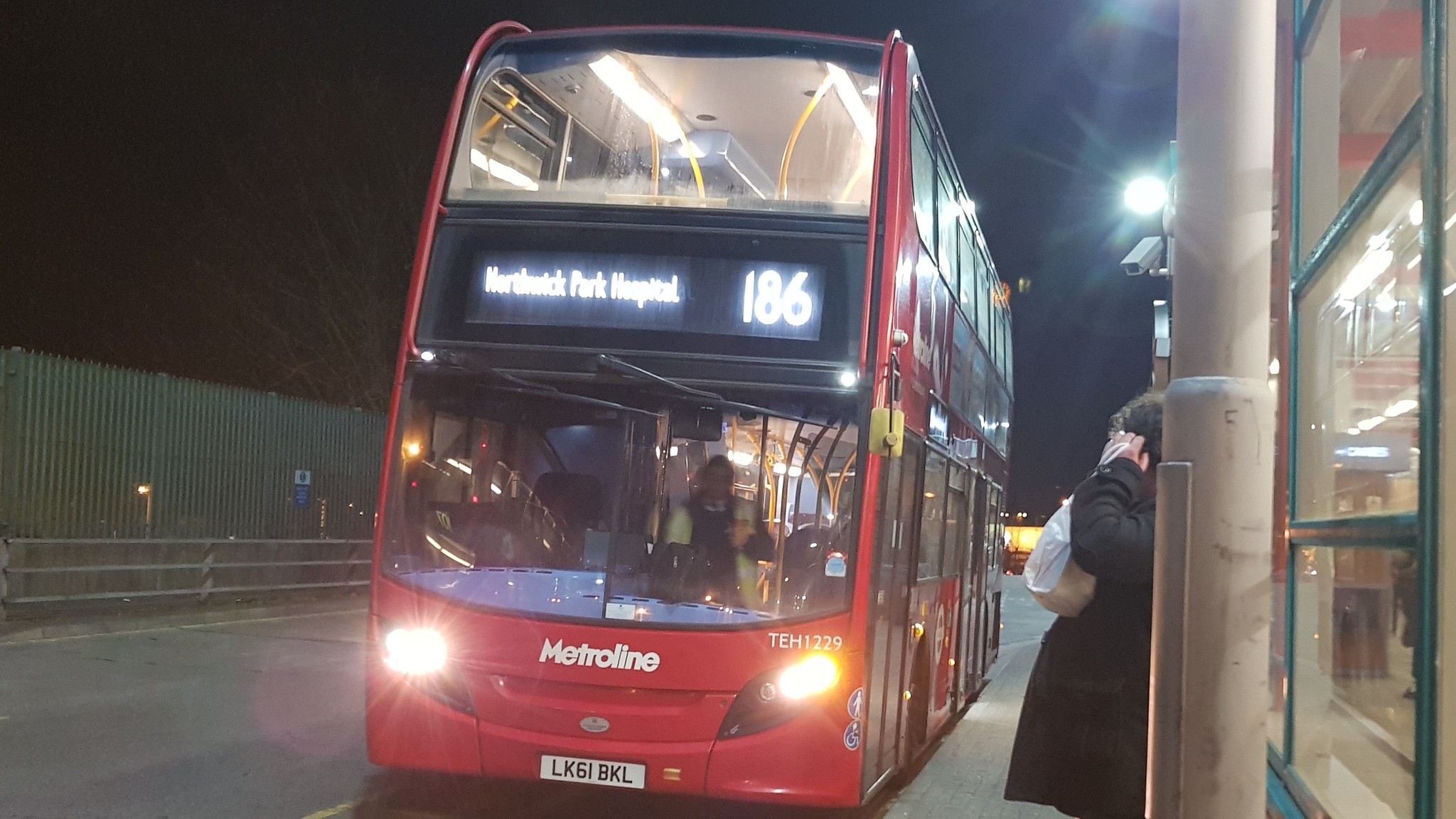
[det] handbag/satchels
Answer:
[1019,442,1129,618]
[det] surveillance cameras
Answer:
[1120,236,1164,275]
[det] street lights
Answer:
[137,484,153,538]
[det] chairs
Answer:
[520,471,601,538]
[775,521,841,616]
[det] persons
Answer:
[1004,393,1161,819]
[664,455,774,610]
[1392,546,1420,699]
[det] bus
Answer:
[361,18,1020,817]
